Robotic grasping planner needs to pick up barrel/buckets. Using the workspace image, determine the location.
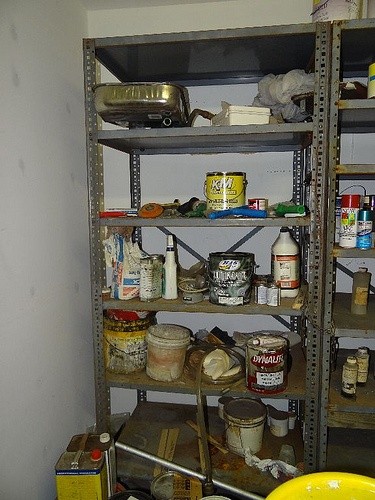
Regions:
[102,317,151,375]
[203,251,256,307]
[145,323,191,382]
[202,170,248,213]
[244,334,289,397]
[55,432,117,500]
[218,396,297,456]
[112,471,232,500]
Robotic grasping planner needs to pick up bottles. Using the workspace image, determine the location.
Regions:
[351,267,371,315]
[341,356,358,398]
[254,281,281,307]
[269,227,300,298]
[355,348,369,388]
[334,194,375,251]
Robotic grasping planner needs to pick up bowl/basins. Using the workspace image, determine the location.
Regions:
[185,345,245,385]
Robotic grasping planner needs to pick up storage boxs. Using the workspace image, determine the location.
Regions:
[212,106,271,125]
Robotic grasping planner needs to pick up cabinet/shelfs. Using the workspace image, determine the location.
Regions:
[82,18,375,500]
